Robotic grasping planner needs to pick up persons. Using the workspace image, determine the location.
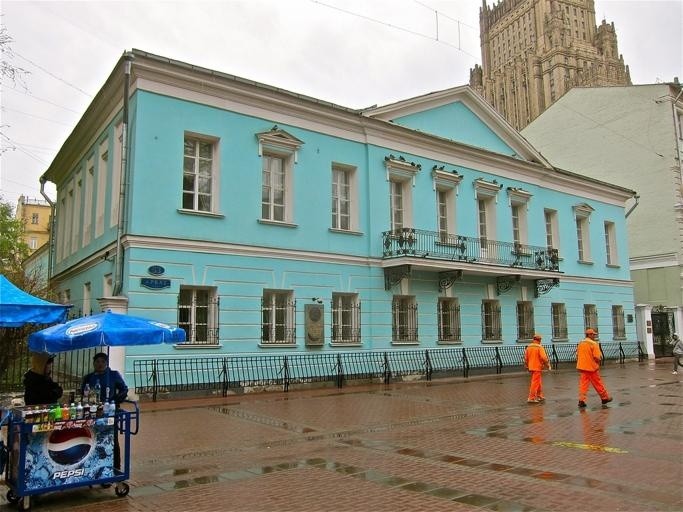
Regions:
[525,335,551,403]
[667,333,683,374]
[24,353,64,405]
[81,352,128,469]
[576,329,614,404]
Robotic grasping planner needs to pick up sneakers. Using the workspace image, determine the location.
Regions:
[537,397,546,401]
[672,371,678,374]
[527,398,540,403]
[602,398,613,404]
[578,401,586,406]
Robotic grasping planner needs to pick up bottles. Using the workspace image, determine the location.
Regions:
[23,398,117,424]
[68,379,102,403]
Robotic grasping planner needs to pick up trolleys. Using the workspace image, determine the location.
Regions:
[0,389,139,512]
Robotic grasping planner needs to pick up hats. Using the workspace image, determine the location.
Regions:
[534,334,543,340]
[93,353,107,361]
[585,329,598,335]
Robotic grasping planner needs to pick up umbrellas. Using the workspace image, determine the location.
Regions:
[28,307,187,354]
